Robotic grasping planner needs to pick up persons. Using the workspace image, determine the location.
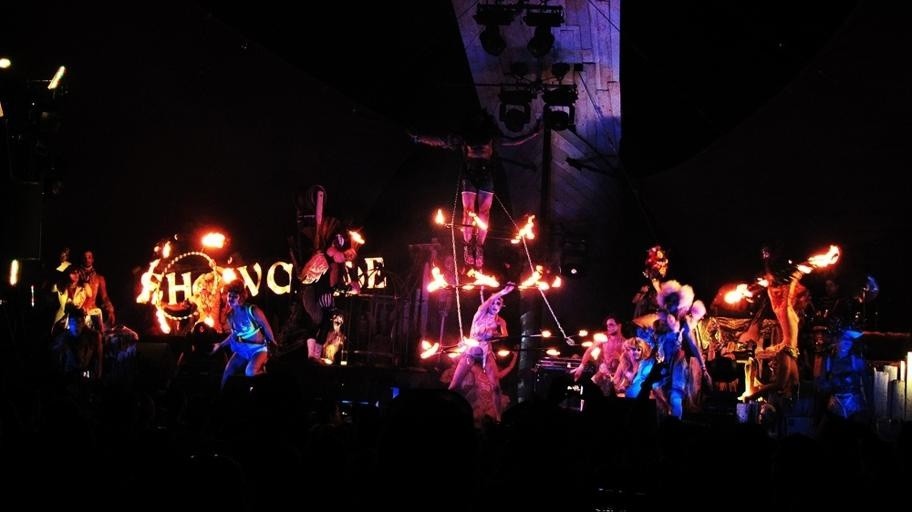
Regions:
[2,225,911,512]
[404,109,542,270]
